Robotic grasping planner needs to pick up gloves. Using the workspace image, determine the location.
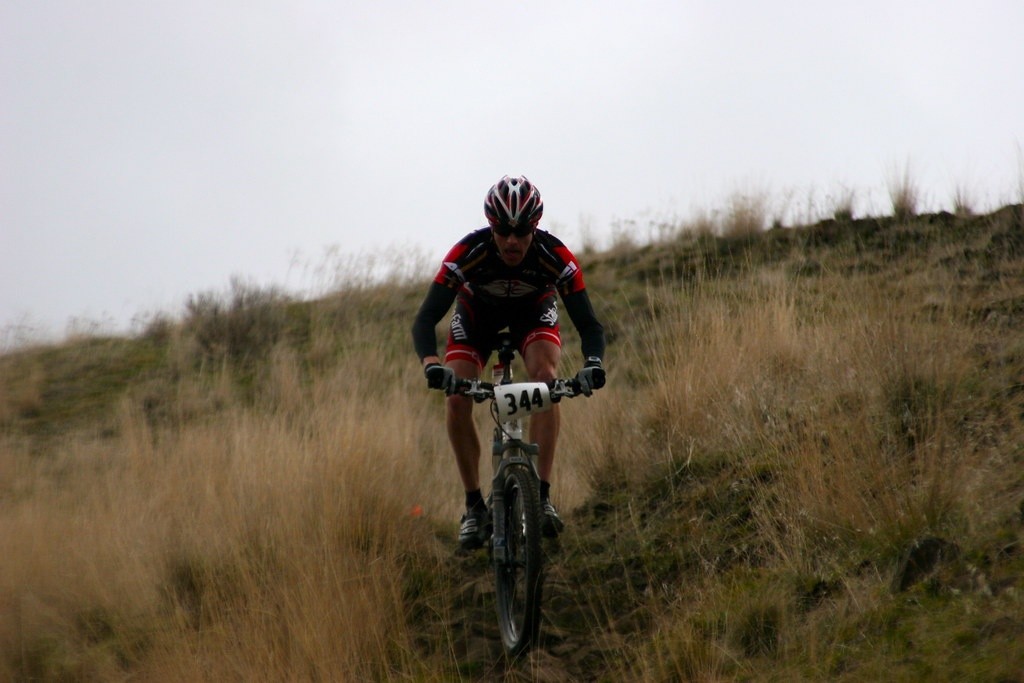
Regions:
[575,360,606,389]
[424,363,450,389]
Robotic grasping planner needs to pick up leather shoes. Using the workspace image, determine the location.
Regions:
[525,498,564,536]
[458,496,491,548]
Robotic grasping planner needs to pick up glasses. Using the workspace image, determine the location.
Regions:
[491,222,535,237]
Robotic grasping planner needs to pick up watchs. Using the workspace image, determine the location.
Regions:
[584,356,602,369]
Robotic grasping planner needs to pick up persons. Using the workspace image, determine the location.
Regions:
[412,175,607,548]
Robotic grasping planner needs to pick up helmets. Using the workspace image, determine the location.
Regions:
[484,175,543,227]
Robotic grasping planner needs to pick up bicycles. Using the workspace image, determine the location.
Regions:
[439,375,584,655]
